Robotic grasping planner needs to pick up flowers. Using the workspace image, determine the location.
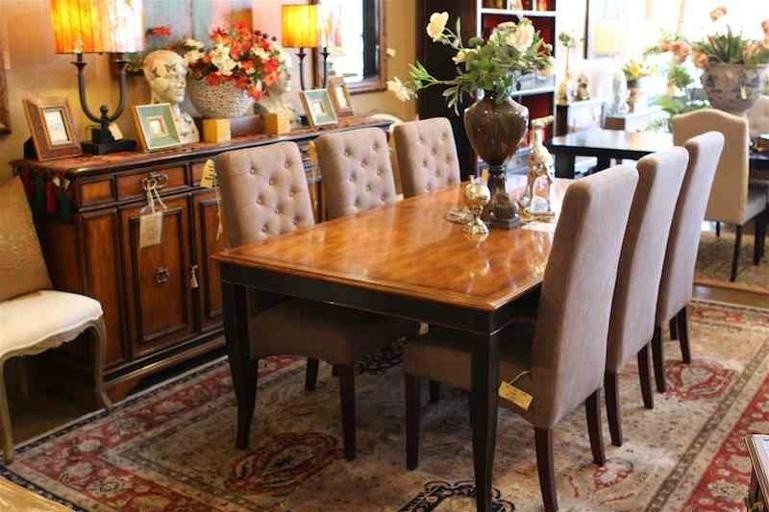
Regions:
[142,22,290,102]
[645,6,769,72]
[386,11,553,116]
[623,61,656,79]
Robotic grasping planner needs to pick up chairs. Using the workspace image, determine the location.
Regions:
[649,130,725,395]
[672,108,767,283]
[600,144,695,447]
[394,115,460,197]
[318,127,397,376]
[1,175,116,468]
[214,140,419,462]
[402,160,644,503]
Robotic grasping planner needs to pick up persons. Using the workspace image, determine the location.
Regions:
[143,49,200,145]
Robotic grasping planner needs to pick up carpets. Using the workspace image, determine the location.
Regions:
[1,276,768,508]
[693,224,769,295]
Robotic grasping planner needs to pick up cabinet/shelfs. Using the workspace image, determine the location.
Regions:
[384,0,555,180]
[9,125,388,390]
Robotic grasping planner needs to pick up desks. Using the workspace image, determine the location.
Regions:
[542,129,769,256]
[556,100,609,136]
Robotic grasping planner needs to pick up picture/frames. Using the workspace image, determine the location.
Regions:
[299,88,338,128]
[22,97,84,162]
[129,102,181,154]
[329,77,353,117]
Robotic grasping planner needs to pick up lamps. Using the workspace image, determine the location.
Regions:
[50,0,145,155]
[281,4,330,89]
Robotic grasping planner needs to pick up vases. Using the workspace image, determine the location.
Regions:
[699,61,762,116]
[463,86,529,232]
